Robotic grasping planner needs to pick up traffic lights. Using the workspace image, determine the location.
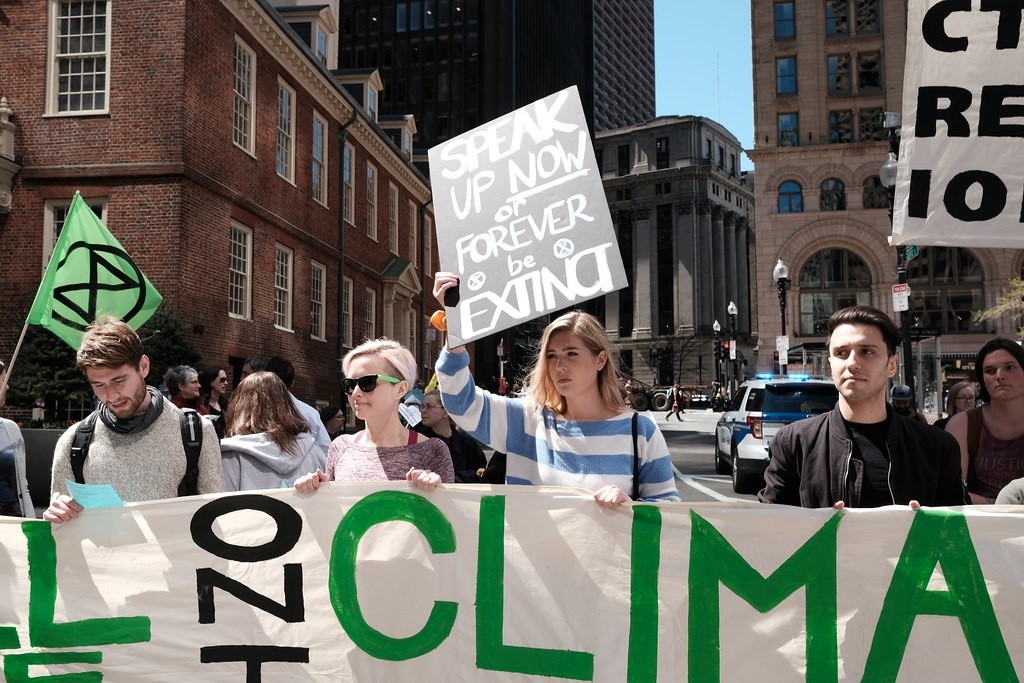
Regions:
[723,342,729,359]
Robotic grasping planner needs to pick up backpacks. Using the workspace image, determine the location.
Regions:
[667,387,673,401]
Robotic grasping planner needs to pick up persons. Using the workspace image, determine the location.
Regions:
[159,352,506,484]
[294,335,455,492]
[0,359,38,518]
[618,377,636,410]
[890,378,978,430]
[432,270,681,509]
[43,312,226,523]
[945,337,1024,505]
[220,371,327,492]
[711,377,725,404]
[665,381,686,422]
[758,305,965,508]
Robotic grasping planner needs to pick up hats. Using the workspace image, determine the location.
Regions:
[320,405,340,424]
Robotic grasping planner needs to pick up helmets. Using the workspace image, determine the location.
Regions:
[892,385,912,400]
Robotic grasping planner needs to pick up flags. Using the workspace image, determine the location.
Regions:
[26,190,164,352]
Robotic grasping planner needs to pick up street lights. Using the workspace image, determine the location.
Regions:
[880,128,918,413]
[728,301,738,394]
[713,320,722,389]
[773,258,791,375]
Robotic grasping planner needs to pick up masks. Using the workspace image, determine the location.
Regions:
[893,399,911,417]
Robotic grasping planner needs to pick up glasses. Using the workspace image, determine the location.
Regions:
[954,397,975,403]
[216,377,228,383]
[419,403,442,412]
[341,374,401,396]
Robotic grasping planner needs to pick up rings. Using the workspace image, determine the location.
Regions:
[427,475,431,480]
[420,469,423,474]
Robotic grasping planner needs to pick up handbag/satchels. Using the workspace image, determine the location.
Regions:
[672,402,678,412]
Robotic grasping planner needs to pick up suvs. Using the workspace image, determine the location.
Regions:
[715,379,841,493]
